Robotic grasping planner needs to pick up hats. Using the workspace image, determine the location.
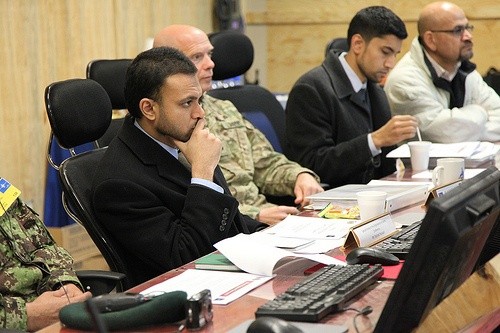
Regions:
[58,291,190,333]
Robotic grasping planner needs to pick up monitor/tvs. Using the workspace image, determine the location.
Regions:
[371,165,500,333]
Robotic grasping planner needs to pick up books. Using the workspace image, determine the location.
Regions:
[195,253,242,271]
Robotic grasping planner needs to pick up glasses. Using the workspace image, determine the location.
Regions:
[431,25,474,38]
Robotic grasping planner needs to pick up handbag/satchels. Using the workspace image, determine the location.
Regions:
[483,67,500,95]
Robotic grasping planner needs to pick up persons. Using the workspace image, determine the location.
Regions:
[92,46,270,285]
[0,177,92,333]
[383,2,500,143]
[285,6,418,191]
[153,24,324,226]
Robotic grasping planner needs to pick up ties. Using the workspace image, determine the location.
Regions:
[358,88,368,106]
[177,150,192,172]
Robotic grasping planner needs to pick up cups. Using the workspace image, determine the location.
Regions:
[432,158,464,187]
[356,191,387,222]
[407,141,432,170]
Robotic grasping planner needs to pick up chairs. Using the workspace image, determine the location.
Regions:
[43,30,348,298]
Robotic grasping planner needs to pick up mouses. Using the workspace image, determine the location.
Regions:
[346,247,399,265]
[246,316,302,333]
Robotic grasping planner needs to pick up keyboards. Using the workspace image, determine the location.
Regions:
[255,264,384,321]
[372,219,423,259]
